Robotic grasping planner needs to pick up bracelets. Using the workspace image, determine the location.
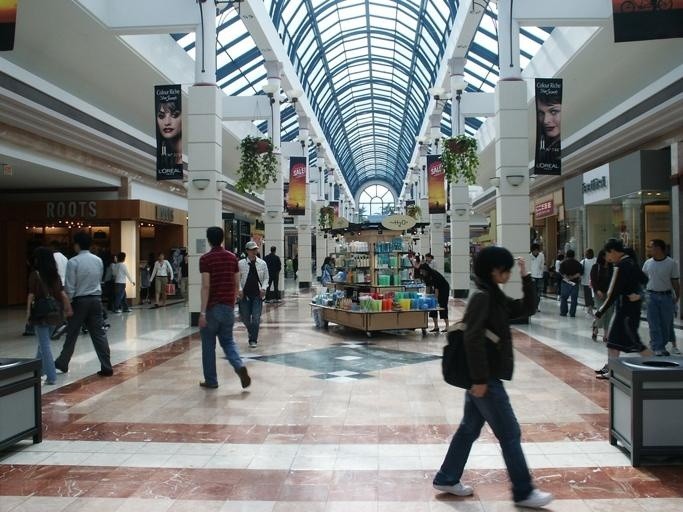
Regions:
[201,312,206,316]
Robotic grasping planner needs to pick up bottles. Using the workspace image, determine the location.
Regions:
[349,254,369,283]
[340,297,352,310]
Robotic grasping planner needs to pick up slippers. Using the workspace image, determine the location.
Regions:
[595,373,608,379]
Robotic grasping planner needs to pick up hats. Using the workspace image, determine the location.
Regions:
[245,241,258,250]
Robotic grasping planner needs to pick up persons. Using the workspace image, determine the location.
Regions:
[237,241,269,347]
[580,249,597,306]
[641,239,677,354]
[403,250,450,332]
[537,97,560,151]
[149,252,173,306]
[156,102,182,164]
[26,248,72,384]
[286,255,298,280]
[556,249,583,317]
[198,227,251,389]
[264,246,282,303]
[530,244,545,312]
[432,246,553,509]
[321,257,346,292]
[590,238,652,378]
[51,230,135,377]
[178,253,188,306]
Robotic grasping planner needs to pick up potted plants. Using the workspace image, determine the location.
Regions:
[231,132,278,195]
[404,203,420,222]
[436,132,481,187]
[316,204,336,232]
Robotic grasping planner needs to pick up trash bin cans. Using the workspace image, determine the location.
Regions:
[0,358,42,449]
[607,358,683,467]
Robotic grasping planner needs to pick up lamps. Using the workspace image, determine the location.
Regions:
[506,174,524,186]
[260,81,279,142]
[297,133,343,189]
[453,81,468,130]
[489,176,500,187]
[279,89,302,114]
[403,132,441,189]
[216,180,227,192]
[191,178,208,189]
[428,87,452,111]
[529,177,536,186]
[267,210,278,220]
[182,180,188,190]
[455,209,465,215]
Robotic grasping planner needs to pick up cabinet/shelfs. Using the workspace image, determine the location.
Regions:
[312,242,445,340]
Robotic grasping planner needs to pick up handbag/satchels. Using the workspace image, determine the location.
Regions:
[29,270,62,321]
[588,263,600,288]
[164,284,175,295]
[441,290,503,391]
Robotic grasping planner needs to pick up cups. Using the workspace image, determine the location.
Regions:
[327,299,333,306]
[372,300,382,312]
[399,299,410,310]
[385,242,389,252]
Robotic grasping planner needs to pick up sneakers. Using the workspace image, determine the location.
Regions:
[249,341,257,347]
[199,380,217,388]
[239,366,250,388]
[670,346,679,354]
[433,479,474,496]
[513,487,554,508]
